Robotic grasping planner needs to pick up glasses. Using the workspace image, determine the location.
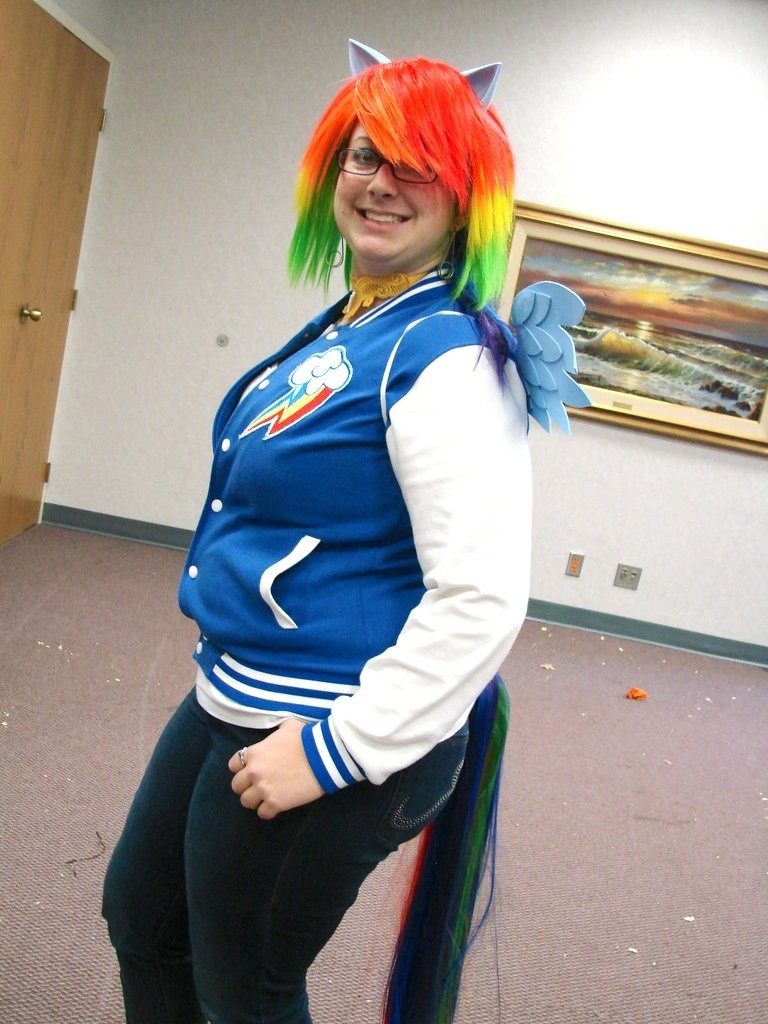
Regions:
[338,148,437,184]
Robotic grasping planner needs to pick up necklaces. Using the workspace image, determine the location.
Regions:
[341,270,429,321]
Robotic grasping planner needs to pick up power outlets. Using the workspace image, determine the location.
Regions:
[613,563,643,591]
[565,551,585,577]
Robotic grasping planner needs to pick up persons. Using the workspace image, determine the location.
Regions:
[94,55,537,1023]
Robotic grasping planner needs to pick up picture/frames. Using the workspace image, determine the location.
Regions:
[488,198,768,459]
